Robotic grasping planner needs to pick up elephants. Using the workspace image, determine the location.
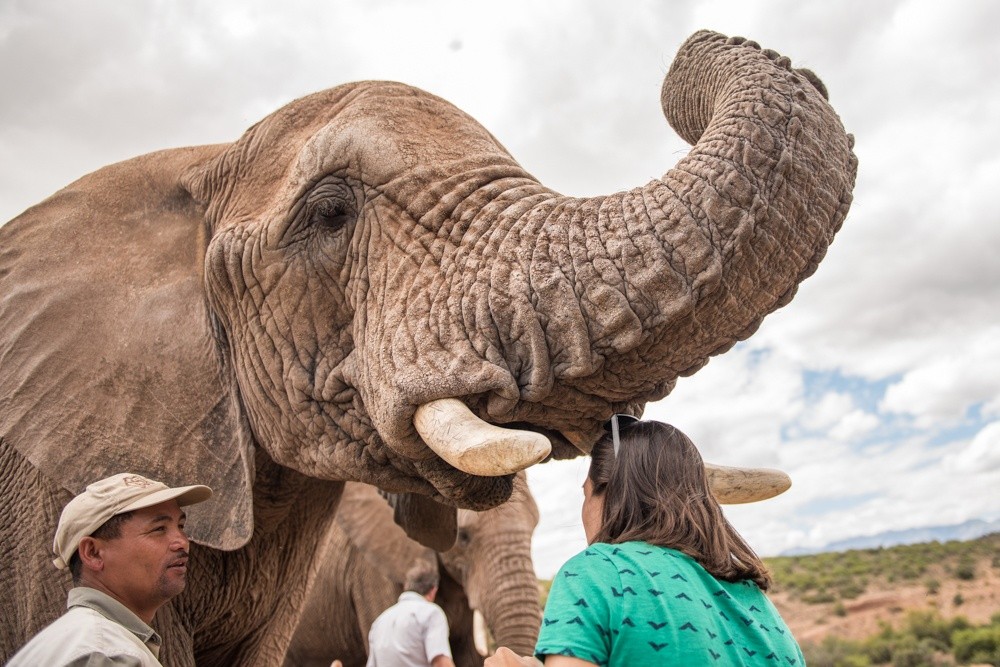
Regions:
[0,29,859,667]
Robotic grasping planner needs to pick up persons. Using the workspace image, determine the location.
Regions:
[366,564,455,667]
[0,473,215,667]
[485,414,804,667]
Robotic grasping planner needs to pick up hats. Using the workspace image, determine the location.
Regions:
[53,470,214,570]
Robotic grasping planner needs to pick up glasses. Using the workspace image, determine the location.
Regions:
[602,413,640,460]
